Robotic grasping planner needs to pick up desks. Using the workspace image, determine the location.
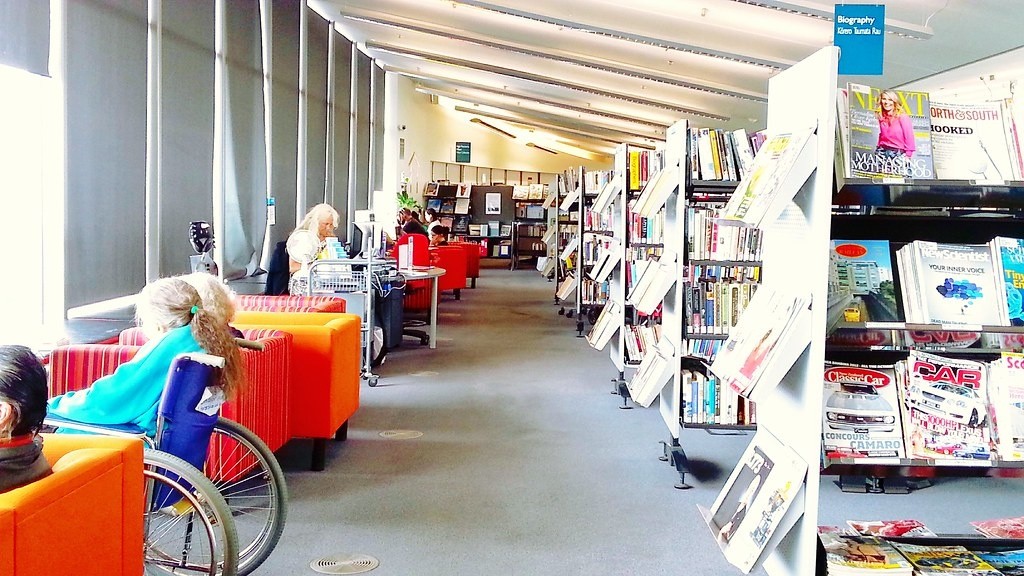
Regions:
[396,266,447,350]
[306,239,447,386]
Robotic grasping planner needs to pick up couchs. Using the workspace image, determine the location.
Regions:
[453,243,481,290]
[391,235,440,311]
[428,245,466,299]
[236,294,346,313]
[228,311,362,472]
[0,433,143,576]
[48,326,293,514]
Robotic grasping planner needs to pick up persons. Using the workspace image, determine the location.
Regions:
[0,345,51,491]
[285,203,340,295]
[871,89,916,176]
[397,201,450,247]
[36,272,245,446]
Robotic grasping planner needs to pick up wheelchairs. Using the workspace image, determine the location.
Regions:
[38,337,289,576]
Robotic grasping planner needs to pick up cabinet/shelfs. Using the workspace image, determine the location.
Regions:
[415,48,1024,576]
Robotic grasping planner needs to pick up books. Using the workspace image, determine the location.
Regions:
[424,173,550,259]
[630,118,772,426]
[553,164,614,308]
[817,83,1024,576]
